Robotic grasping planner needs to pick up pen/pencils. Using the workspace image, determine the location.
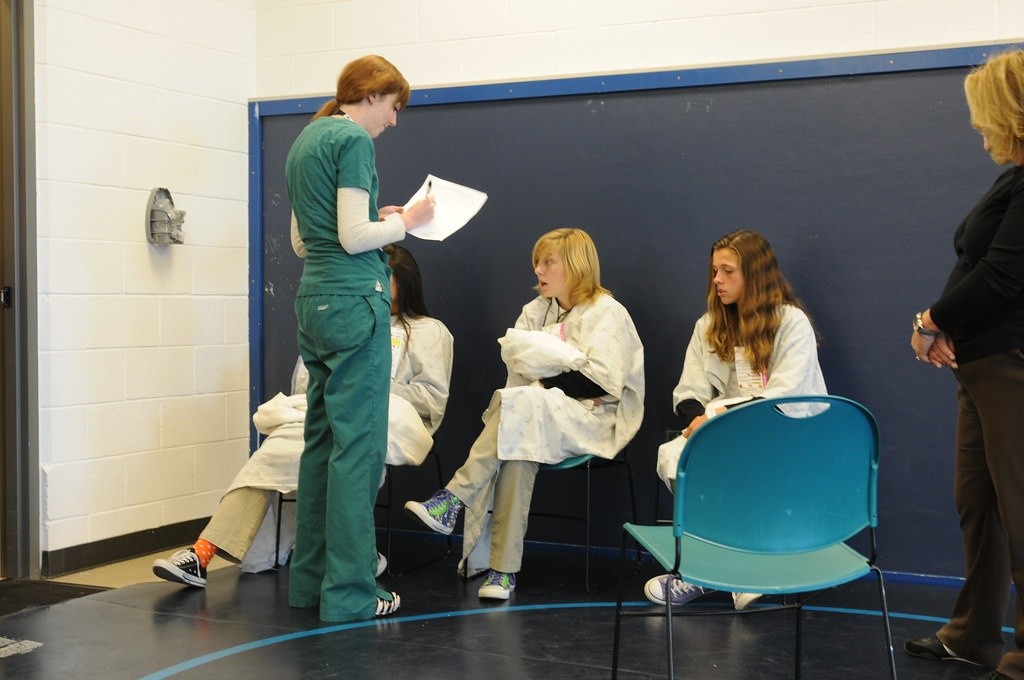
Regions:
[425,180,433,199]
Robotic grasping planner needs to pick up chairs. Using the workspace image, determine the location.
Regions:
[612,396,901,680]
[464,446,643,594]
[274,451,452,578]
[652,429,831,606]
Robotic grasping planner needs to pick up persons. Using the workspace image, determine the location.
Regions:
[644,228,830,609]
[901,50,1024,680]
[404,228,645,599]
[284,54,437,622]
[154,244,454,588]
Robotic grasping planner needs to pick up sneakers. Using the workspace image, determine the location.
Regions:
[477,570,516,600]
[374,551,387,578]
[644,574,716,606]
[978,671,1012,680]
[374,591,400,616]
[152,548,207,589]
[732,591,763,611]
[904,636,987,667]
[404,489,465,536]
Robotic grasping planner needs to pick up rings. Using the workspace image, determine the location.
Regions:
[916,356,920,360]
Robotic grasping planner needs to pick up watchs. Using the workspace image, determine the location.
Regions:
[913,312,936,335]
